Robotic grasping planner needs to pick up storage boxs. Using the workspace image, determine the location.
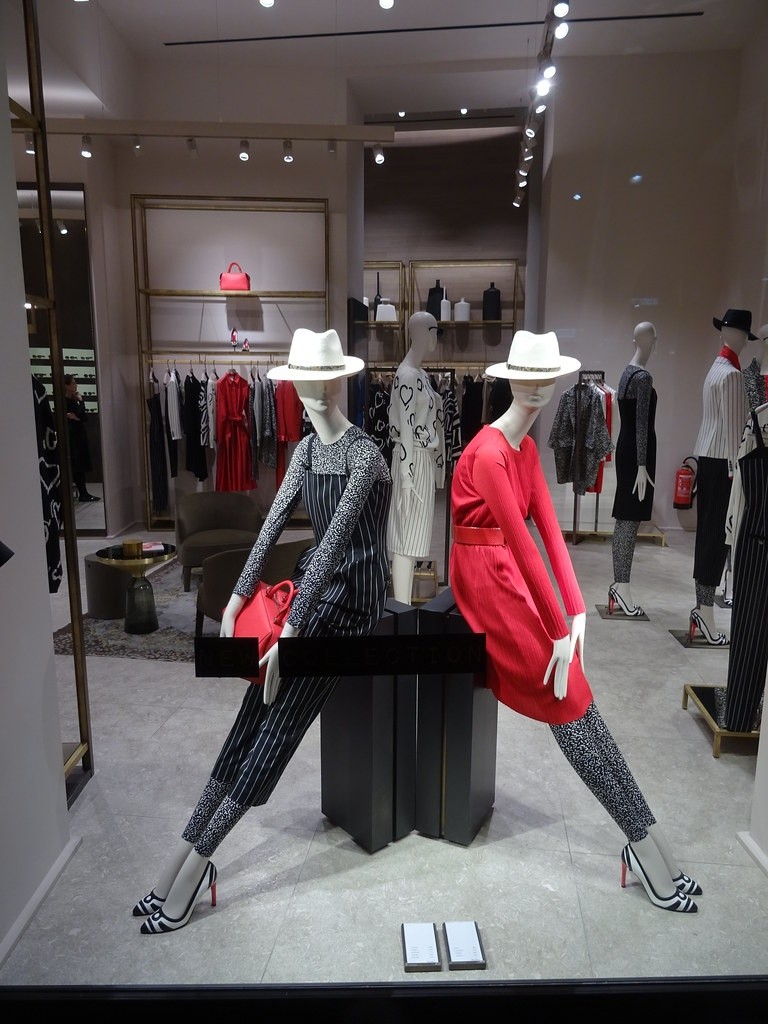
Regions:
[417,582,500,845]
[314,594,413,853]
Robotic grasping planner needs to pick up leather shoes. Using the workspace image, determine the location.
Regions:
[78,494,101,502]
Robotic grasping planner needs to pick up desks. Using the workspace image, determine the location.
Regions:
[98,541,179,636]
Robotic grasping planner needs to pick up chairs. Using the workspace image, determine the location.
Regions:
[191,534,321,638]
[172,489,262,589]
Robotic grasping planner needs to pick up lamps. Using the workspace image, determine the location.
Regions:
[80,135,94,160]
[282,140,294,164]
[550,0,571,19]
[35,220,41,234]
[518,158,534,176]
[522,116,544,138]
[536,51,557,79]
[544,14,570,40]
[513,190,526,208]
[328,140,338,154]
[238,139,250,163]
[23,133,34,156]
[514,169,528,188]
[521,130,539,149]
[520,141,534,161]
[530,79,555,98]
[57,220,68,236]
[186,138,197,155]
[528,88,548,115]
[130,135,147,158]
[373,143,385,167]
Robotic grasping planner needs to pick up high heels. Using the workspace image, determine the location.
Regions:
[609,588,645,616]
[723,569,734,606]
[688,606,730,645]
[230,328,250,352]
[619,844,703,913]
[133,861,217,934]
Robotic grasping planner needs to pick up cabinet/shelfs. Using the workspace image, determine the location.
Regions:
[28,339,99,417]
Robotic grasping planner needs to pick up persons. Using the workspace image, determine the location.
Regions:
[65,374,101,502]
[743,324,768,411]
[450,329,703,913]
[689,308,760,645]
[132,328,394,935]
[608,322,658,616]
[387,312,445,605]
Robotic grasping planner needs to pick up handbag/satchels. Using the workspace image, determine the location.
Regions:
[219,262,251,291]
[221,579,301,685]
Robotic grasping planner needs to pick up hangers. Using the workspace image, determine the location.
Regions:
[371,363,503,383]
[572,370,607,393]
[147,358,286,381]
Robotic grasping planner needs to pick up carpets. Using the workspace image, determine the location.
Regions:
[52,557,222,664]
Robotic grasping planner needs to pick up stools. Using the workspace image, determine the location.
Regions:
[86,552,132,619]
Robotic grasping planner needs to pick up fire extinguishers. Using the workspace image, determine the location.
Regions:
[672,457,700,509]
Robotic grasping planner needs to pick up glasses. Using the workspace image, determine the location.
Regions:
[33,355,44,358]
[428,326,444,337]
[66,374,78,376]
[65,356,77,359]
[83,391,95,395]
[34,374,46,377]
[81,357,92,359]
[84,374,95,377]
[85,409,96,412]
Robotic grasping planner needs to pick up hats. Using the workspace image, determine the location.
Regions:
[484,331,581,381]
[713,309,760,341]
[266,328,365,381]
